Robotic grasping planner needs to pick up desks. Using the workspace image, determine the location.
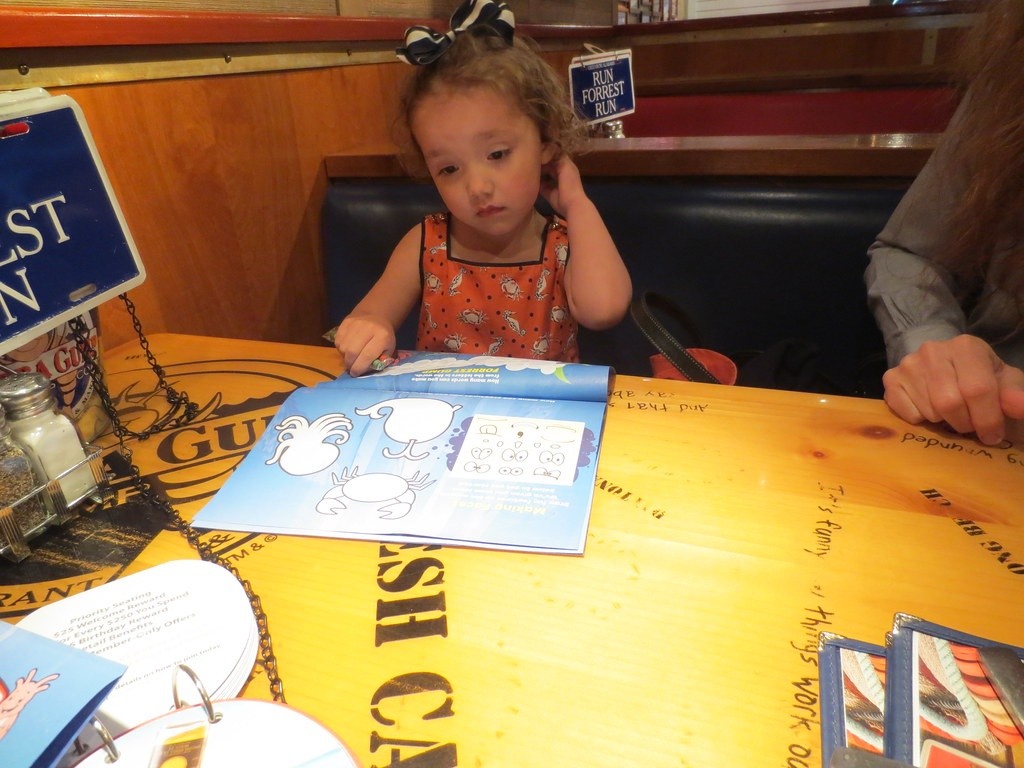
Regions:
[0,334,1024,768]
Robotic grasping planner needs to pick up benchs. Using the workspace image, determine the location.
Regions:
[317,129,992,400]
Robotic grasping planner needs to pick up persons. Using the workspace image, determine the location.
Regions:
[335,0,633,364]
[861,0,1024,446]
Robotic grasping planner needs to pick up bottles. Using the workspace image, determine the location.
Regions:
[605,119,625,139]
[0,373,96,542]
